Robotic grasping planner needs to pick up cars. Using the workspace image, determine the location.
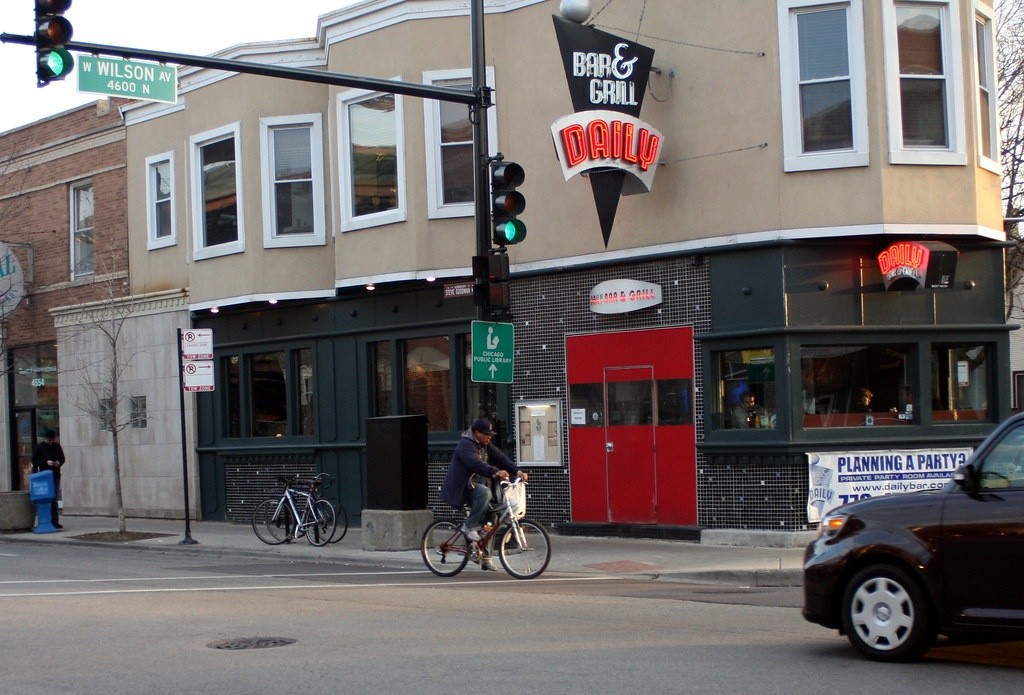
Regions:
[802,412,1024,664]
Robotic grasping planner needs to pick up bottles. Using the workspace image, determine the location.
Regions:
[480,522,492,538]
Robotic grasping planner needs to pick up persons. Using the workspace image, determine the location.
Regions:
[847,390,873,414]
[438,420,530,571]
[31,430,66,529]
[900,387,913,414]
[732,393,757,429]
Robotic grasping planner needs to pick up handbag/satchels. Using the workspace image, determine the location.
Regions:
[502,481,527,524]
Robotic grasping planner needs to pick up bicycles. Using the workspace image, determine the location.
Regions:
[421,475,552,580]
[251,472,349,547]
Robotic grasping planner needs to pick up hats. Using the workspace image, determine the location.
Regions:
[474,418,497,436]
[44,430,57,439]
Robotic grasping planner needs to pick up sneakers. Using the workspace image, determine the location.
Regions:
[461,523,481,542]
[481,560,498,571]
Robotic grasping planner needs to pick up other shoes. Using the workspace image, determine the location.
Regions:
[54,523,63,529]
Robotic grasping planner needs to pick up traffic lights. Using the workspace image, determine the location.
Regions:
[472,251,511,315]
[35,0,75,81]
[491,162,527,246]
